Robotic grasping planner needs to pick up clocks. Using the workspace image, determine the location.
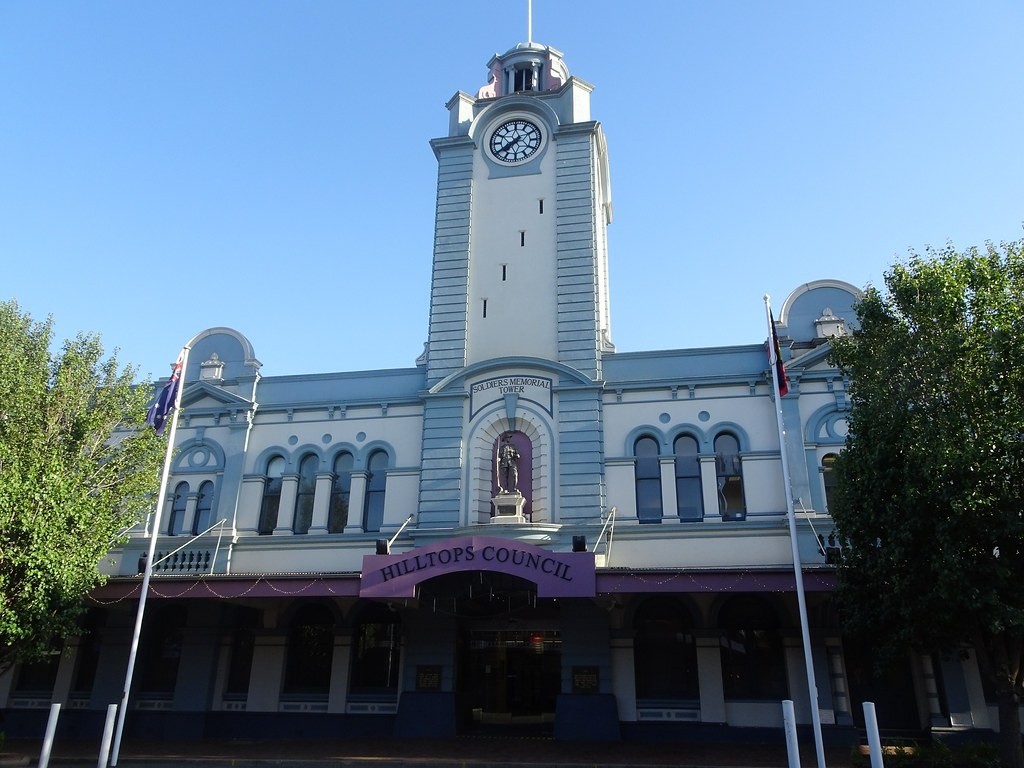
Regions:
[482,111,548,167]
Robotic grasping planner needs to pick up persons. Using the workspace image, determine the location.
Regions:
[496,433,520,493]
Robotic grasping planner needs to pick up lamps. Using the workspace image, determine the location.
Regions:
[376,516,412,556]
[572,536,587,552]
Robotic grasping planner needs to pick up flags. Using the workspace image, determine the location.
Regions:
[147,362,183,437]
[768,309,788,397]
